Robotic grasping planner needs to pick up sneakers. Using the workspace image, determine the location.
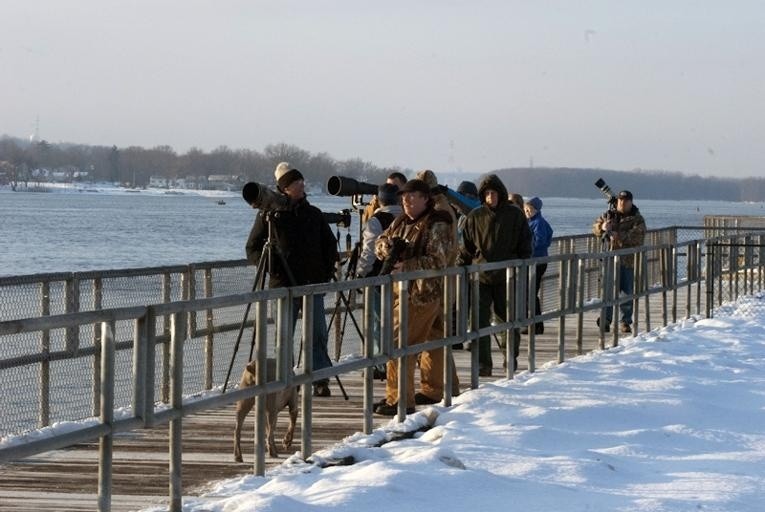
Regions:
[416,393,438,405]
[522,327,544,335]
[375,405,415,415]
[479,369,492,376]
[596,318,610,332]
[312,385,332,398]
[621,323,632,333]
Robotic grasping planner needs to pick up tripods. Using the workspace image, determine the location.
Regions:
[218,240,380,400]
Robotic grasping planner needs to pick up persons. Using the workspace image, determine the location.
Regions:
[243,160,338,398]
[349,168,554,378]
[370,179,455,417]
[591,188,647,336]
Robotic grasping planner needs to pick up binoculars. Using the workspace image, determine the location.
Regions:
[220,182,353,399]
[594,178,618,241]
[327,176,385,397]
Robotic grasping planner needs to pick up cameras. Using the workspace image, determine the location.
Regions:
[594,176,616,202]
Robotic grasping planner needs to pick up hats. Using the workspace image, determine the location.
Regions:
[414,170,438,188]
[526,197,543,211]
[274,161,305,190]
[456,181,478,195]
[396,179,429,195]
[615,190,634,201]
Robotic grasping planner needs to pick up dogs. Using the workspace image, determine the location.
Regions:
[233,358,298,463]
